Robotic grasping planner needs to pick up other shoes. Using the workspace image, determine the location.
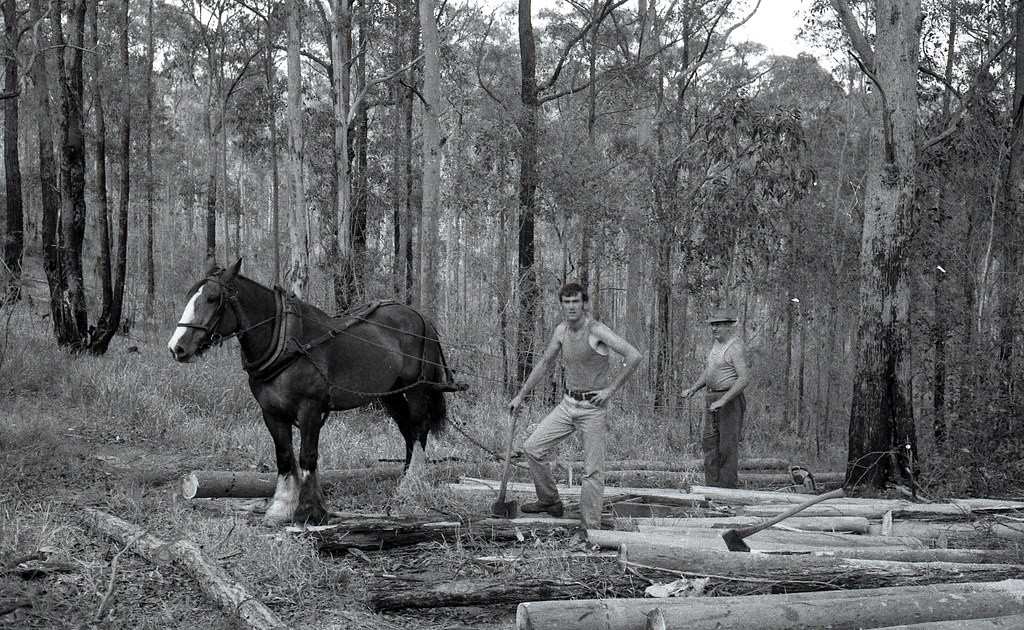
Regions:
[521,500,564,518]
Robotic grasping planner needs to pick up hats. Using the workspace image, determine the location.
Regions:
[705,309,737,326]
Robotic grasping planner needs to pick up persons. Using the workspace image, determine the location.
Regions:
[509,282,643,540]
[680,317,750,489]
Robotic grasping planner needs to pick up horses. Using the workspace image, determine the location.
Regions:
[166,256,447,527]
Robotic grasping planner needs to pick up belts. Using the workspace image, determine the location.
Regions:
[565,388,598,401]
[707,388,730,394]
